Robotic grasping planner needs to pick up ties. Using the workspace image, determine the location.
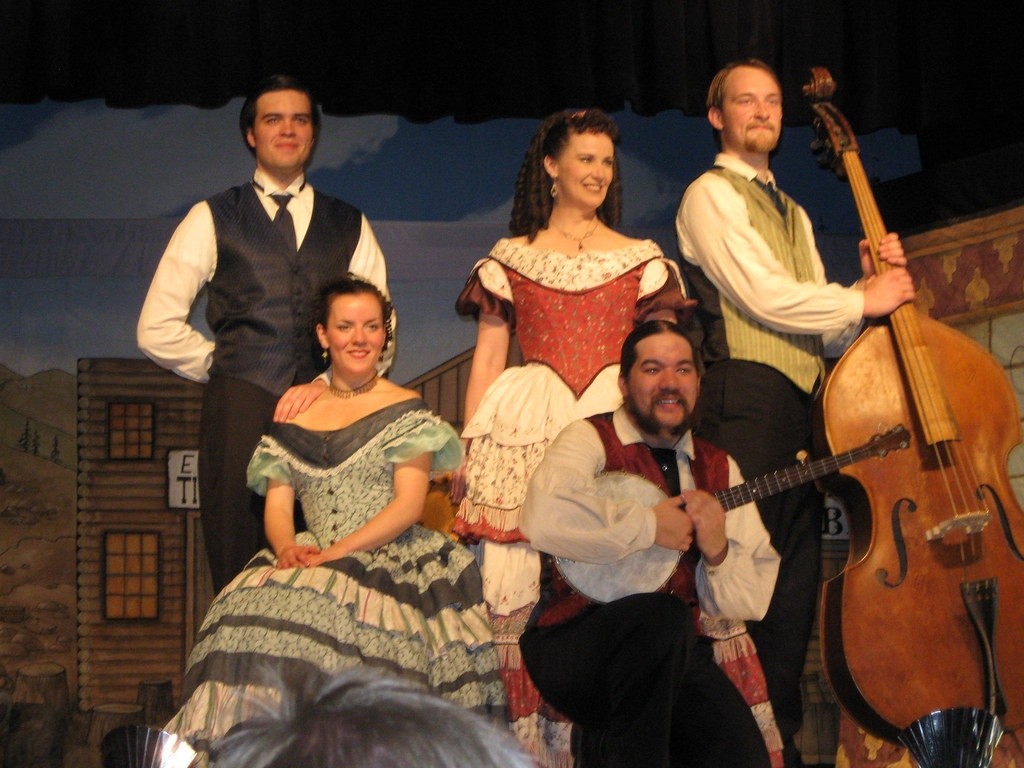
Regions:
[708,161,788,218]
[251,171,307,255]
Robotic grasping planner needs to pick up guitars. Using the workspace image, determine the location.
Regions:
[547,421,916,608]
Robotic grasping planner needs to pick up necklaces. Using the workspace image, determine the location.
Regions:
[549,215,600,250]
[330,370,379,399]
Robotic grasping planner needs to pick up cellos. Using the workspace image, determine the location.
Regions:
[799,58,1024,746]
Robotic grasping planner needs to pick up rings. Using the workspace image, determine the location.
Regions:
[680,493,688,503]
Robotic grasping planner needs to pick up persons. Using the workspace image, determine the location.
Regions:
[162,273,507,768]
[208,661,534,768]
[675,59,916,768]
[519,321,782,768]
[137,73,395,595]
[450,107,783,768]
